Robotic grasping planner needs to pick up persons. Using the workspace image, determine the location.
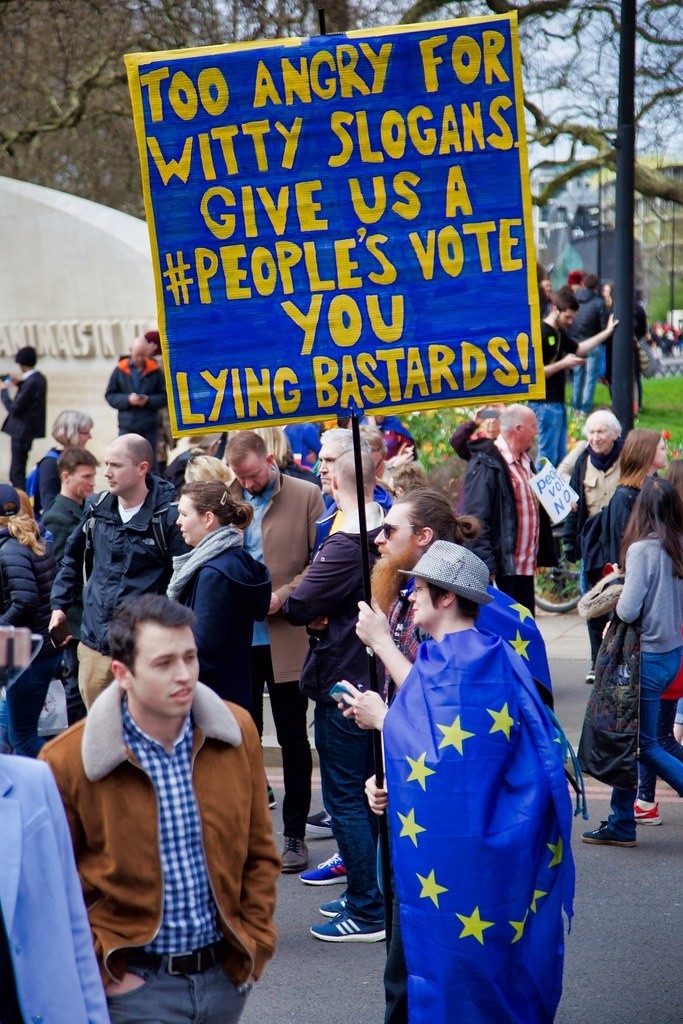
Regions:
[0,407,550,942]
[647,322,683,357]
[0,346,47,489]
[0,752,110,1024]
[338,540,590,1023]
[38,594,282,1024]
[536,262,648,468]
[106,331,176,476]
[557,409,683,847]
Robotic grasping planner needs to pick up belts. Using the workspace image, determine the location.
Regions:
[124,941,229,976]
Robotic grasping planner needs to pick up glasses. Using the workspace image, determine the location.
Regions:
[380,524,416,540]
[412,584,430,592]
[657,445,670,453]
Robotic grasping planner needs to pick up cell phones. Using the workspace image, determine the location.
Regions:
[328,682,355,707]
[480,410,499,419]
[0,628,33,669]
[49,620,71,649]
[138,394,144,399]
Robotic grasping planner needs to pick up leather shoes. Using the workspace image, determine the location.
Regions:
[280,837,309,874]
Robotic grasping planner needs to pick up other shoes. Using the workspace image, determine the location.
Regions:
[266,786,277,809]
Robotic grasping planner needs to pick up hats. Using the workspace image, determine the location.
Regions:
[144,330,163,357]
[0,483,21,516]
[15,346,37,368]
[396,540,496,606]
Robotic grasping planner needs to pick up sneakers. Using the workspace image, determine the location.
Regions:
[310,910,387,943]
[305,809,333,836]
[298,851,348,885]
[319,896,348,918]
[633,798,662,826]
[580,820,638,847]
[585,663,596,684]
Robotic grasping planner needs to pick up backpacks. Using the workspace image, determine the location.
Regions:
[25,451,60,520]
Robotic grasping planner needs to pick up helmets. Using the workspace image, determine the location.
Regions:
[567,269,588,286]
